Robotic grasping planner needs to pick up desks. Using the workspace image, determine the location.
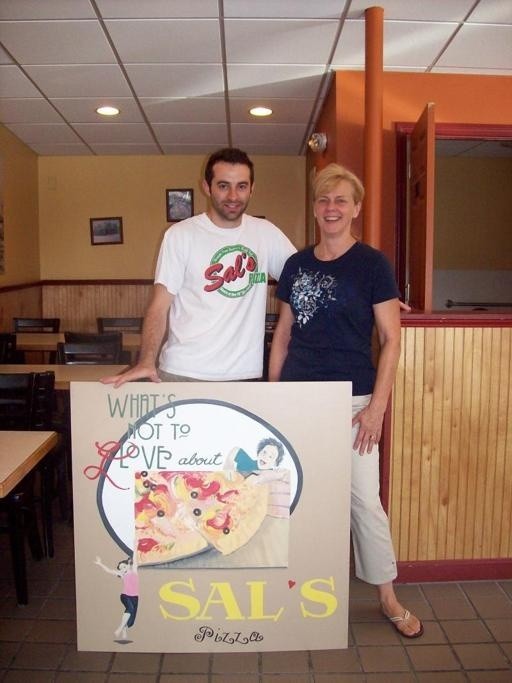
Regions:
[0,428,60,609]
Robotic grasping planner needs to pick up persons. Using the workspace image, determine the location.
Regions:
[268,161,424,642]
[93,535,140,640]
[221,437,284,482]
[99,145,415,390]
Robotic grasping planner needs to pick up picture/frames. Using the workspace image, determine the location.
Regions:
[89,216,123,245]
[165,188,195,222]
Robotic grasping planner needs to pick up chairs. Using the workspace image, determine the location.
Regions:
[1,316,145,560]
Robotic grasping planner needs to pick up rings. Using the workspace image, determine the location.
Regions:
[369,434,377,439]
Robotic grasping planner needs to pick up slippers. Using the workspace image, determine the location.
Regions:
[385,609,424,639]
